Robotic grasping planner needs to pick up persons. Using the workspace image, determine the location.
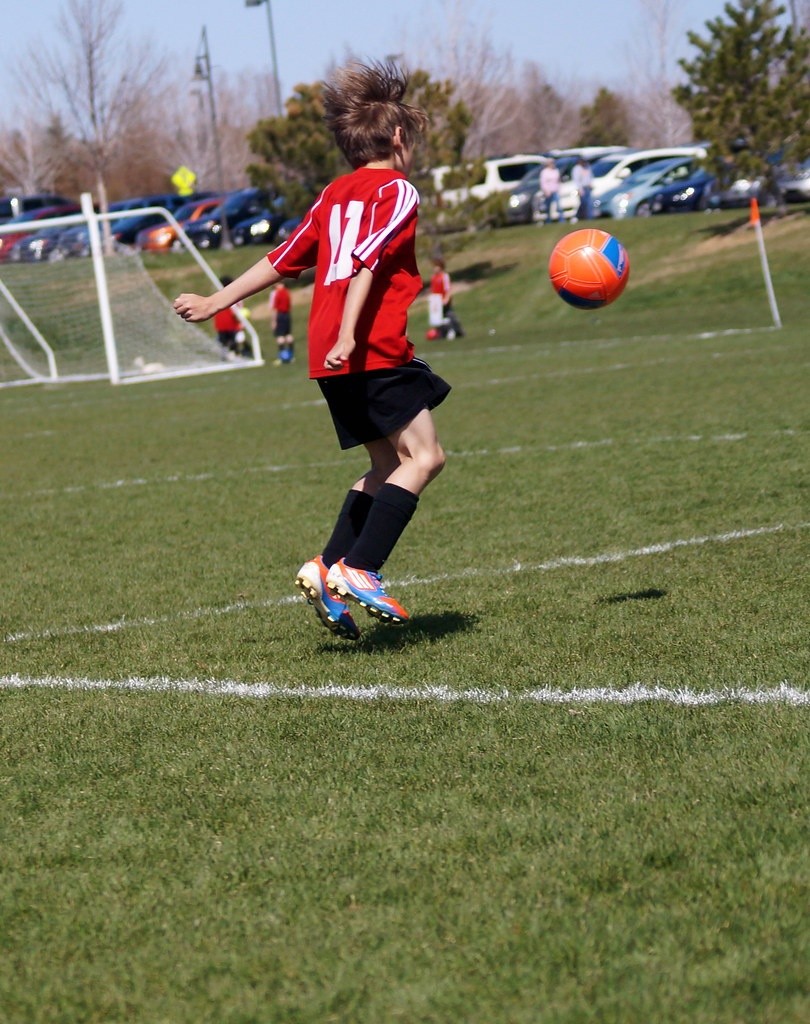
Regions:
[171,57,452,638]
[269,282,299,368]
[425,258,457,341]
[571,157,594,220]
[213,277,247,360]
[539,158,566,223]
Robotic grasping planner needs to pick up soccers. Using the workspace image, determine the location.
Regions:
[549,228,630,311]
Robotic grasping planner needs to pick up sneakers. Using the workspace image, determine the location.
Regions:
[295,554,359,641]
[325,557,409,625]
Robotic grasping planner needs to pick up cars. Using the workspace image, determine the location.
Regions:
[0,186,302,262]
[410,141,810,219]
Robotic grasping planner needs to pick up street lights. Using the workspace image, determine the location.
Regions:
[192,25,234,251]
[246,0,282,117]
